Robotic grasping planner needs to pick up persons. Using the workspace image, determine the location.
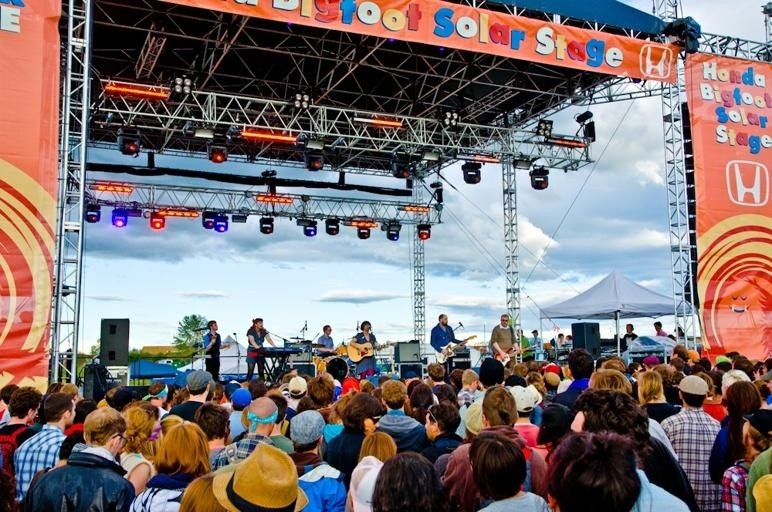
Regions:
[128,421,212,512]
[59,383,81,401]
[668,357,684,372]
[287,375,307,409]
[490,313,523,379]
[636,371,682,425]
[653,320,668,336]
[660,375,722,512]
[224,381,242,413]
[349,320,384,381]
[305,374,335,411]
[628,361,645,377]
[720,411,772,512]
[366,452,449,512]
[697,355,724,395]
[517,329,533,363]
[97,384,133,411]
[746,445,772,511]
[245,317,277,386]
[287,409,347,512]
[194,402,233,460]
[179,473,227,512]
[323,391,388,492]
[171,367,213,422]
[504,361,529,389]
[570,388,654,470]
[697,371,728,422]
[25,429,86,512]
[444,385,550,512]
[425,403,465,463]
[201,319,231,383]
[45,383,63,393]
[148,381,169,419]
[543,370,561,405]
[565,335,573,345]
[374,379,428,450]
[467,431,551,511]
[326,357,350,402]
[316,324,338,358]
[708,381,771,486]
[429,313,467,378]
[622,323,638,350]
[122,400,156,419]
[0,385,43,512]
[509,384,544,447]
[210,397,277,474]
[671,344,689,365]
[0,383,19,424]
[555,332,571,351]
[588,368,679,462]
[349,430,398,512]
[528,329,542,360]
[211,383,227,402]
[673,326,685,339]
[64,398,98,436]
[120,406,155,512]
[731,357,754,381]
[13,392,77,512]
[536,402,575,446]
[227,387,253,440]
[544,429,691,512]
[641,355,661,369]
[28,407,136,512]
[549,348,596,402]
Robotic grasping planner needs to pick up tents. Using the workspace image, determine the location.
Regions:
[538,267,698,362]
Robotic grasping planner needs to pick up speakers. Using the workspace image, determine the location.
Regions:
[571,321,602,362]
[99,318,130,367]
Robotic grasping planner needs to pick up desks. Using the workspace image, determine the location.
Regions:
[256,347,301,383]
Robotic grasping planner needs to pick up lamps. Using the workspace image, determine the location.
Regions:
[308,155,325,170]
[119,134,141,155]
[512,158,532,170]
[172,77,193,97]
[303,222,317,236]
[392,158,410,177]
[84,204,101,223]
[417,224,432,240]
[358,227,371,240]
[462,162,481,183]
[260,218,274,233]
[536,120,554,136]
[384,221,401,240]
[150,212,166,229]
[325,219,340,235]
[442,109,457,128]
[208,144,228,163]
[112,209,127,225]
[530,167,550,191]
[292,92,311,109]
[201,212,217,230]
[214,216,228,231]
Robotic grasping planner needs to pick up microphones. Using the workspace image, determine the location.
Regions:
[232,332,242,344]
[457,321,464,327]
[259,328,269,334]
[368,325,373,334]
[304,318,308,331]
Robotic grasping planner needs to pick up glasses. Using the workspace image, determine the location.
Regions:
[502,319,508,322]
[370,417,380,425]
[113,433,128,444]
[425,404,435,420]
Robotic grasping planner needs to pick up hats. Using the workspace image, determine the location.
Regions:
[511,384,543,413]
[742,409,772,440]
[544,362,561,377]
[643,357,661,365]
[229,389,252,407]
[672,375,709,395]
[288,375,308,396]
[688,350,701,364]
[349,455,384,512]
[289,410,326,443]
[716,354,733,365]
[761,369,772,380]
[210,444,310,512]
[465,400,483,435]
[545,372,560,386]
[185,368,212,391]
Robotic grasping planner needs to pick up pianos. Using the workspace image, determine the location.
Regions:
[254,346,303,358]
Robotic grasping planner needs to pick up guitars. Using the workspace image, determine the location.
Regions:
[347,340,391,363]
[435,335,477,364]
[494,344,537,368]
